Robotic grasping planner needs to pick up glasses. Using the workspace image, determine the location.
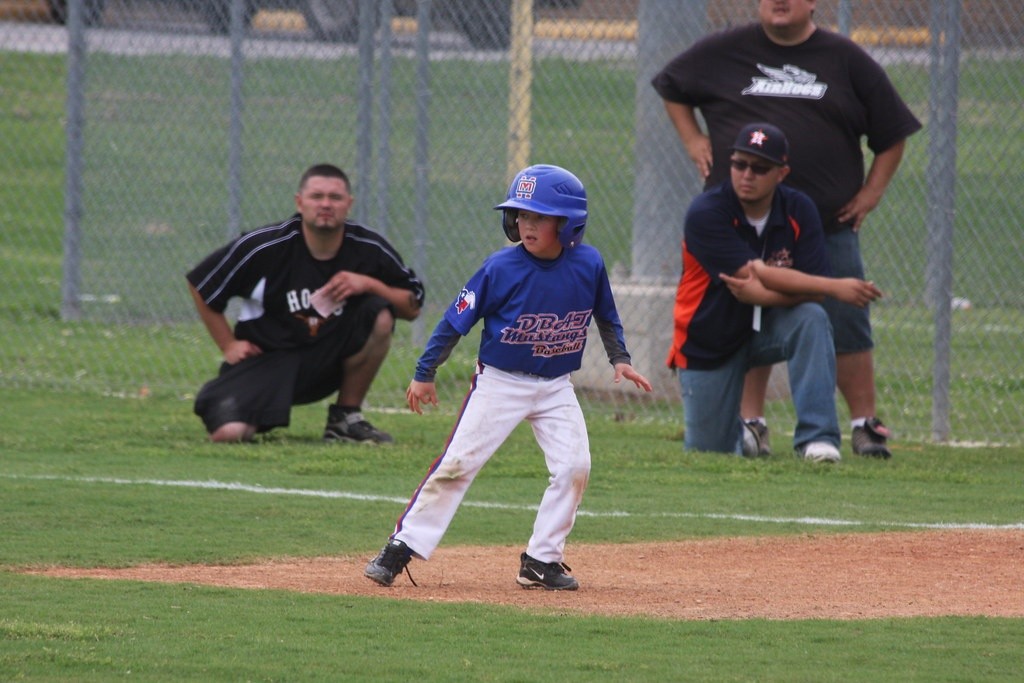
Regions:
[730,155,776,174]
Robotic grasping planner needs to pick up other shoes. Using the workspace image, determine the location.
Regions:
[851,417,892,458]
[747,419,771,456]
[800,441,841,465]
[739,415,761,458]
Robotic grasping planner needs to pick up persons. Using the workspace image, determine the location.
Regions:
[187,165,423,444]
[364,163,649,589]
[651,0,923,460]
[665,125,883,463]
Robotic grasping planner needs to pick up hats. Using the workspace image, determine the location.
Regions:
[732,124,790,166]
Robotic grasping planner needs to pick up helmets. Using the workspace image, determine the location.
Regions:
[493,164,588,249]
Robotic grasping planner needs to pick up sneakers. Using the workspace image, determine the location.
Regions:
[364,537,418,587]
[516,552,581,591]
[323,403,394,449]
